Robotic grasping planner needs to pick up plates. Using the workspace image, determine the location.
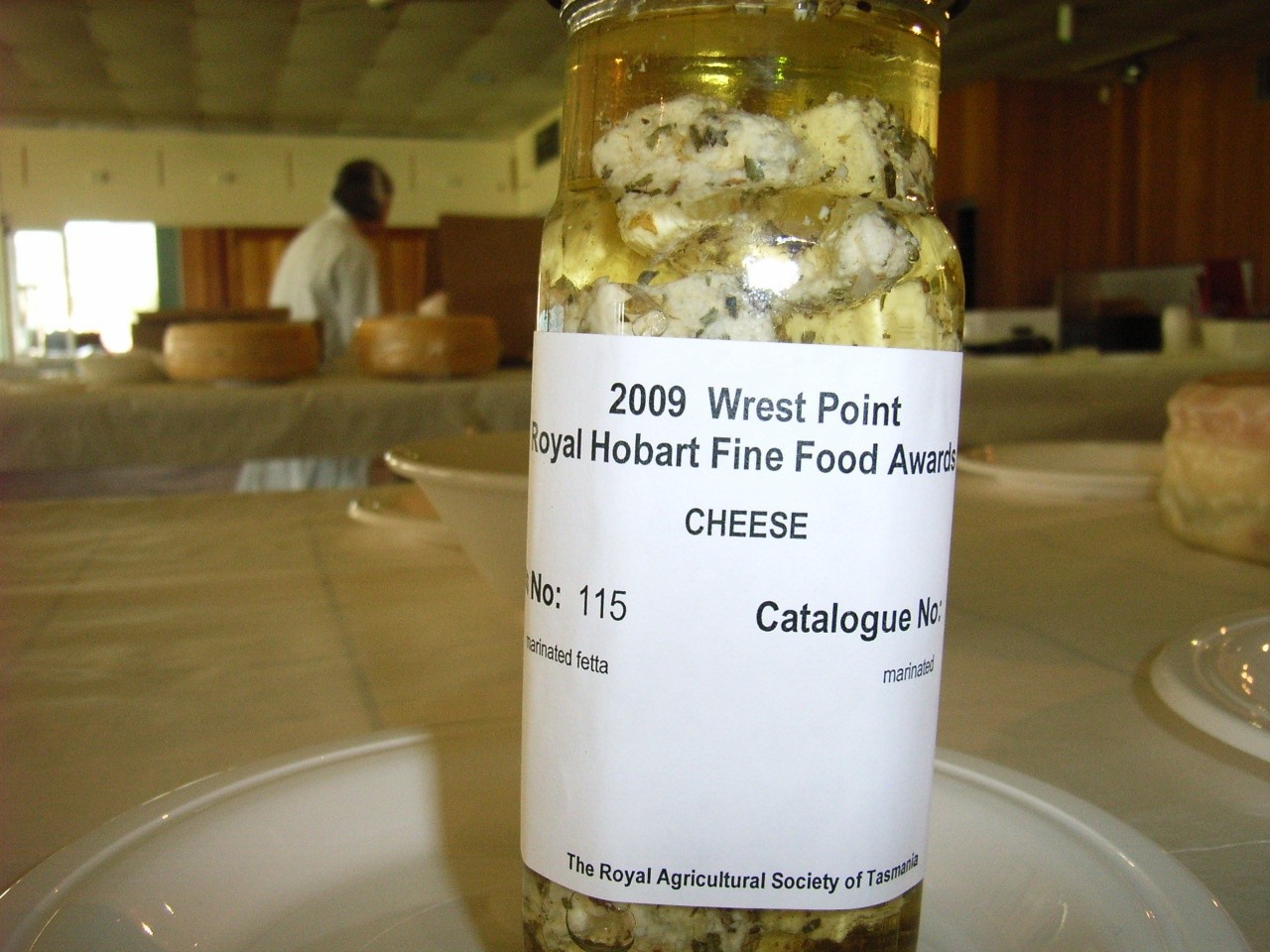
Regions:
[0,713,1250,952]
[955,440,1161,500]
[347,483,459,545]
[1149,606,1270,763]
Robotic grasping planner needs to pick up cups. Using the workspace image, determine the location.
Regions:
[1163,307,1192,352]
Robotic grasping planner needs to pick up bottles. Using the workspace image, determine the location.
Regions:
[517,1,967,952]
[21,330,47,380]
[76,334,112,383]
[42,332,78,380]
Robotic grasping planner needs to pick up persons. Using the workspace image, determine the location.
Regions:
[235,159,393,491]
[416,283,471,319]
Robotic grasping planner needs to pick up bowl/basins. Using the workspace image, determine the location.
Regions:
[163,322,325,383]
[360,317,501,381]
[386,433,529,597]
[1202,320,1270,368]
